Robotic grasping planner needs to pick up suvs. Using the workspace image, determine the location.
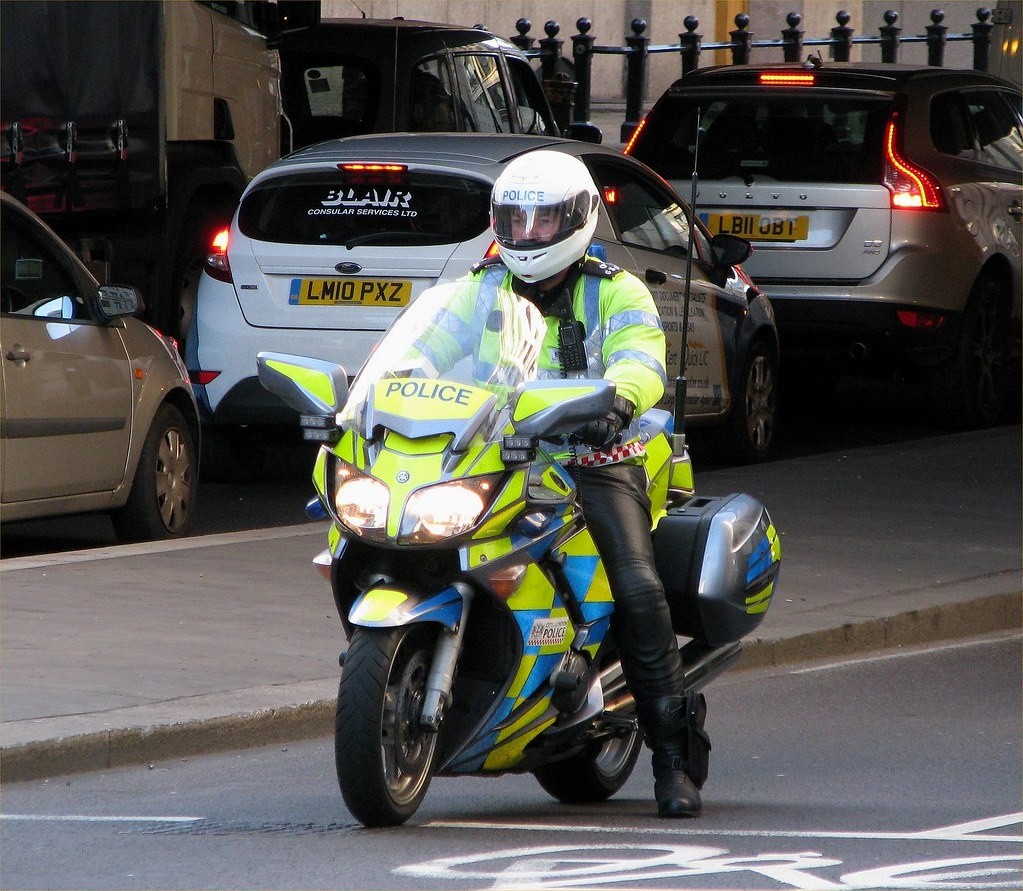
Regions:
[611,57,1023,431]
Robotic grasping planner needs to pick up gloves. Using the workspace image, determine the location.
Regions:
[568,394,636,452]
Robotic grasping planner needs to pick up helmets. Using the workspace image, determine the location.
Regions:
[489,149,600,284]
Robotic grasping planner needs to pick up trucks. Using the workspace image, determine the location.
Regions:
[1,1,294,322]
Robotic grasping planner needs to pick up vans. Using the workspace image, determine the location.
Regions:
[274,16,607,160]
[1,187,202,547]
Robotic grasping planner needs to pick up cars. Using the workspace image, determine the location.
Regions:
[186,130,783,491]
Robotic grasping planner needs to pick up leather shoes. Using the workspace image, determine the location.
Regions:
[651,752,703,818]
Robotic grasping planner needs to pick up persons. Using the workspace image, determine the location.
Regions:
[378,151,712,818]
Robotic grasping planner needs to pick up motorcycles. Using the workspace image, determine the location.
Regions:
[255,277,781,830]
